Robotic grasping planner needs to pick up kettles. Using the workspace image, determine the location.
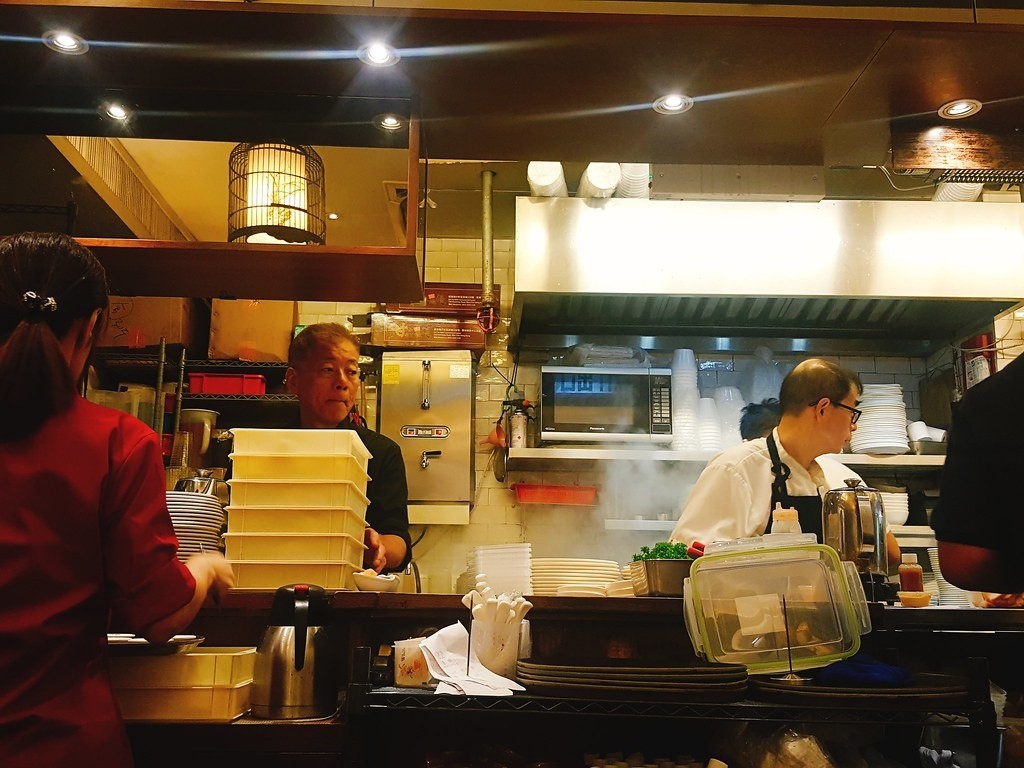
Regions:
[252,584,349,722]
[823,479,898,605]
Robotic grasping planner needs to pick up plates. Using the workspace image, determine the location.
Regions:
[849,383,908,452]
[928,547,972,608]
[516,664,748,695]
[165,491,223,564]
[619,164,650,198]
[466,543,534,596]
[531,558,635,597]
[932,181,984,202]
[577,161,621,197]
[528,161,567,197]
[755,672,976,707]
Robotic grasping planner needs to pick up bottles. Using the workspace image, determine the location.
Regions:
[772,502,804,534]
[897,553,924,593]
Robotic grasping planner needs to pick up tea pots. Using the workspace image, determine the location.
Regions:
[175,470,230,504]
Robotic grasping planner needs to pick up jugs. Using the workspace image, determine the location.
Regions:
[178,410,220,469]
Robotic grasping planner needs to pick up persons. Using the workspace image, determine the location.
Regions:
[0,232,235,768]
[207,322,413,574]
[930,351,1024,594]
[667,358,900,577]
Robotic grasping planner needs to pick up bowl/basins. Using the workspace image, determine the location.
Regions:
[672,348,699,447]
[716,384,753,448]
[354,572,399,591]
[882,492,910,525]
[699,399,721,452]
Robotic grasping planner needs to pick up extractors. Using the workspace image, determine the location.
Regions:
[510,200,1024,356]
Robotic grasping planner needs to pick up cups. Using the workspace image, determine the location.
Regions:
[166,430,193,469]
[470,621,519,682]
[394,641,429,686]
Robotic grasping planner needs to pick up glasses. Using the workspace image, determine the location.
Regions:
[808,400,862,424]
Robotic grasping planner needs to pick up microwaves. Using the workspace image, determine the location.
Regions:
[539,363,673,445]
